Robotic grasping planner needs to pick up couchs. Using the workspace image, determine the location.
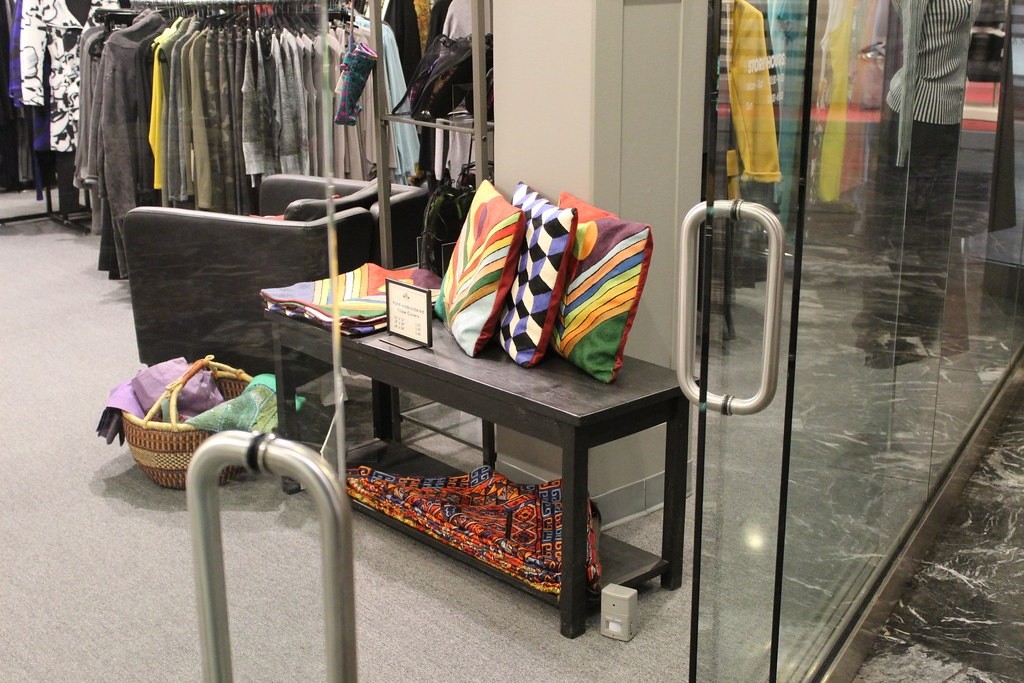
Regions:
[124,166,425,389]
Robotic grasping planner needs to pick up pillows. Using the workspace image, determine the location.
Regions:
[496,182,576,367]
[432,179,527,355]
[550,192,653,384]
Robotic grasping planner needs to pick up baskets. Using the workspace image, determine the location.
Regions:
[120,355,273,489]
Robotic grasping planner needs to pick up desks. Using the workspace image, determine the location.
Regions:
[263,266,698,640]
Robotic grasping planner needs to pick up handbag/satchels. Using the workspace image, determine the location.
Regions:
[417,161,494,280]
[391,33,493,122]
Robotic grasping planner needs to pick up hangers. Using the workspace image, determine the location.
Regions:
[131,0,357,36]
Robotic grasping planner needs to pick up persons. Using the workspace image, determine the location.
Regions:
[858,1,973,367]
